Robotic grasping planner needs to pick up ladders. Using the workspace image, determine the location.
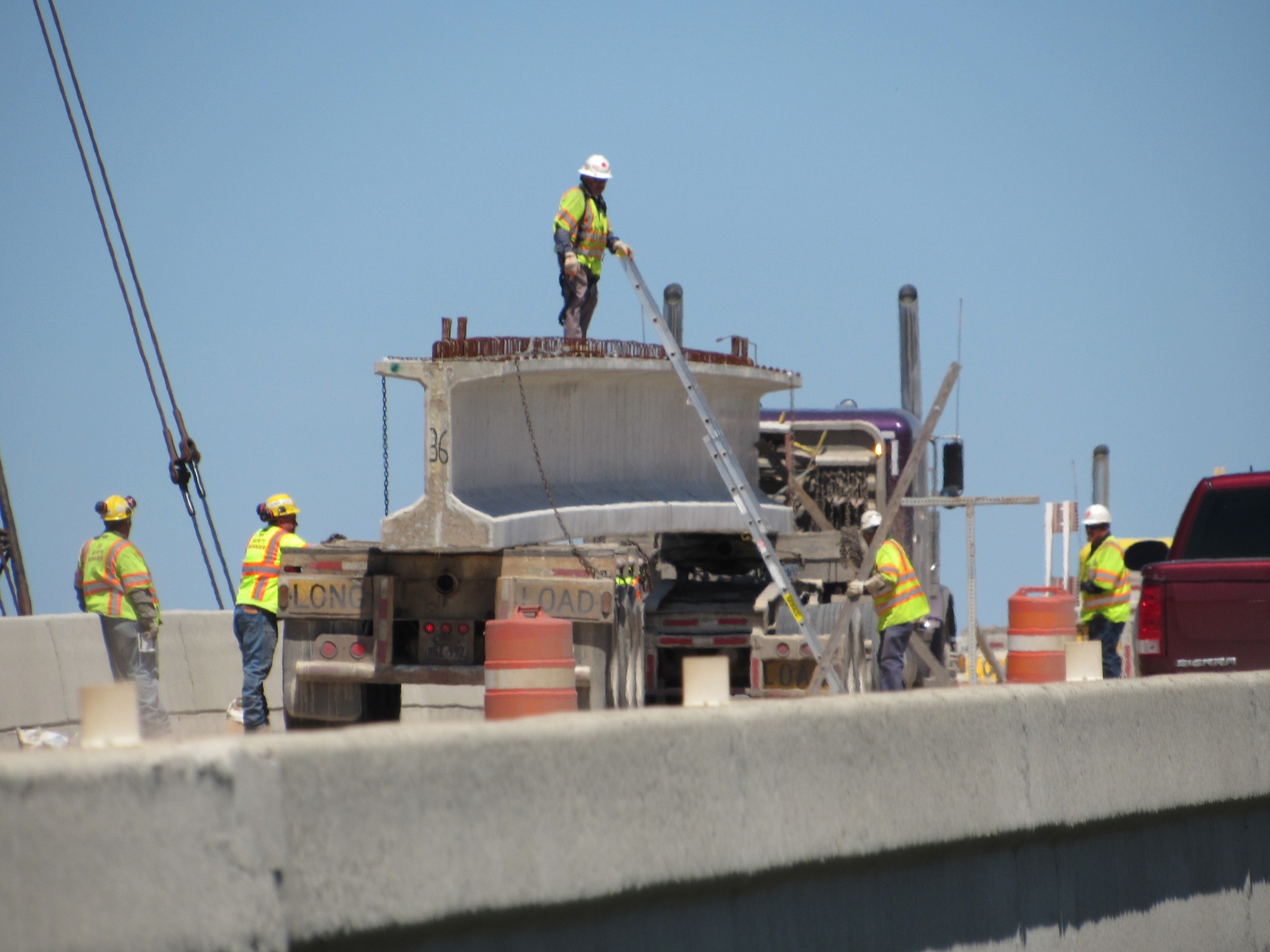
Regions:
[613,246,847,695]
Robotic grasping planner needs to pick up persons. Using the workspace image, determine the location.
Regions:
[75,495,176,745]
[553,154,633,345]
[1079,504,1131,679]
[846,510,930,692]
[234,493,311,735]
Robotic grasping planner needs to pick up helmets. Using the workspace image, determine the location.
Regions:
[101,496,134,522]
[265,495,300,517]
[1082,504,1112,526]
[579,154,613,179]
[858,510,883,532]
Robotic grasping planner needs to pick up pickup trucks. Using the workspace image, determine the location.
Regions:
[1123,469,1270,677]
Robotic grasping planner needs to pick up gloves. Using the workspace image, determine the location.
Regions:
[613,240,633,261]
[794,579,825,593]
[563,251,580,278]
[844,579,863,602]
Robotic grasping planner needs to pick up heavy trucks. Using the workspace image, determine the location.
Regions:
[277,408,967,737]
